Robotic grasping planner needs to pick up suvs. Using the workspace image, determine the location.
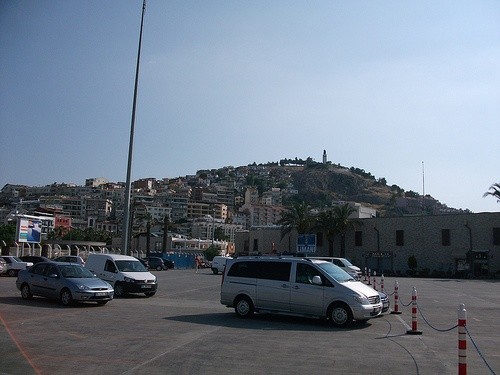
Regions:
[144,257,164,271]
[16,261,114,306]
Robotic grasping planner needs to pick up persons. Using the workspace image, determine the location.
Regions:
[194,254,200,272]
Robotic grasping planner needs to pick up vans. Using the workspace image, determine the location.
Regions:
[305,256,362,280]
[220,256,383,325]
[211,255,233,274]
[85,252,158,297]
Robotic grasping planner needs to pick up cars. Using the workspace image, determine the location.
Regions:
[0,258,7,274]
[376,289,390,314]
[18,256,53,264]
[0,255,33,276]
[163,257,174,269]
[52,255,85,266]
